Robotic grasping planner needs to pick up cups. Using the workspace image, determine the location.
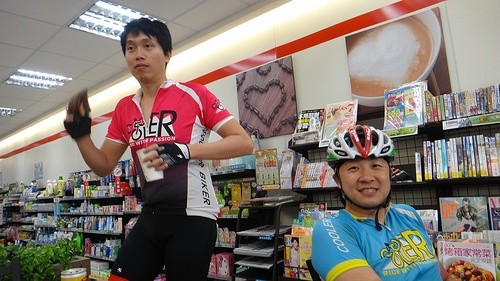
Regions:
[136,145,164,182]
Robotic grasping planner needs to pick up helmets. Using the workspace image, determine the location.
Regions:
[326,124,396,161]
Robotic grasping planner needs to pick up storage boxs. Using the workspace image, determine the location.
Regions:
[54,255,91,276]
[208,251,234,277]
[90,260,112,280]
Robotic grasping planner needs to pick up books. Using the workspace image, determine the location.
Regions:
[414,84,500,281]
[284,98,358,281]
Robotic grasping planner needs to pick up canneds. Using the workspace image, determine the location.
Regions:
[61,268,88,281]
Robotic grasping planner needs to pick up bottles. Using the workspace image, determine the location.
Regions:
[47,176,64,196]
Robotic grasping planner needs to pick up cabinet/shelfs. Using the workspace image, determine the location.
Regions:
[207,169,255,281]
[288,112,500,192]
[232,199,301,281]
[0,175,142,262]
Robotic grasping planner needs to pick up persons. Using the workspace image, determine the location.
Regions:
[64,17,254,281]
[457,199,479,232]
[311,125,455,281]
[290,237,299,272]
